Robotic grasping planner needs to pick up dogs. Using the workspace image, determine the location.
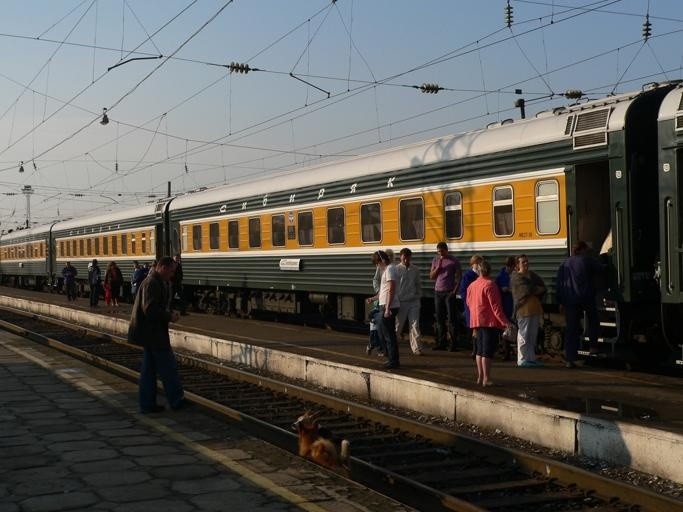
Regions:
[291,410,333,439]
[298,420,338,471]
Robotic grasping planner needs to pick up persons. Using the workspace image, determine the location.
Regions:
[367,242,608,388]
[127,256,195,413]
[61,253,187,316]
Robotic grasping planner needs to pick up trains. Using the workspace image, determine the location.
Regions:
[0,78,682,376]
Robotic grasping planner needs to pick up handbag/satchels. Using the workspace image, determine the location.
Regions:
[503,327,517,343]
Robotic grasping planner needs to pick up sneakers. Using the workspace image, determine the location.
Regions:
[431,344,456,352]
[142,405,164,414]
[522,360,544,368]
[565,360,576,368]
[415,350,423,356]
[377,349,400,369]
[172,398,192,409]
